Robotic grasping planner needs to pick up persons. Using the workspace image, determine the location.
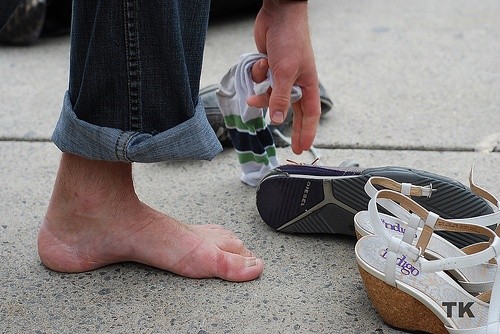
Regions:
[38,1,321,282]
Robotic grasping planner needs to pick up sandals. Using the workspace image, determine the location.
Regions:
[354,167,500,334]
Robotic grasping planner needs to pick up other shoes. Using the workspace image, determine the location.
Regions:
[194,68,335,144]
[255,163,498,250]
